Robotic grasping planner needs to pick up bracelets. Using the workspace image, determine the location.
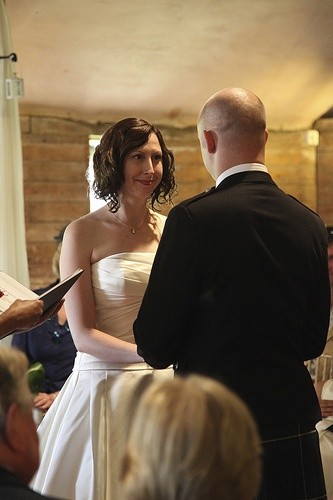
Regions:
[51,392,58,397]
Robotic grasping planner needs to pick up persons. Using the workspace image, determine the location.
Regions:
[11,225,79,427]
[133,86,332,500]
[28,118,175,500]
[110,374,261,499]
[0,299,70,500]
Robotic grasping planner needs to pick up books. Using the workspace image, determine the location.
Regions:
[0,268,85,315]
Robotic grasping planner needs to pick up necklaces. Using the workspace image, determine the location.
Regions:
[109,202,147,234]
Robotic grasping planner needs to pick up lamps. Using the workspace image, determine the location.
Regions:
[0,52,25,101]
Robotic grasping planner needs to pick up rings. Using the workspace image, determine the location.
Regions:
[46,314,54,321]
[44,398,47,401]
